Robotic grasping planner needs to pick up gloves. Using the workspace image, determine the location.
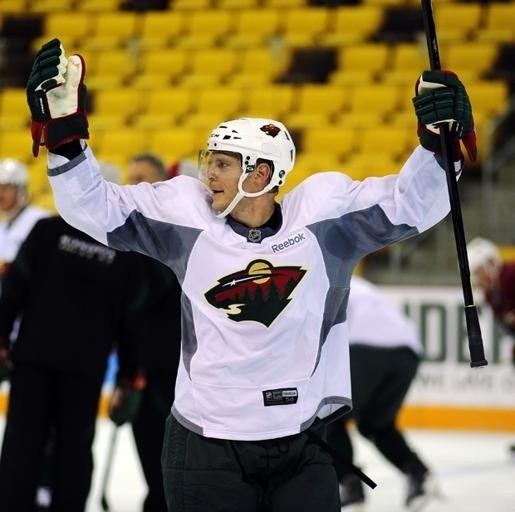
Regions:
[108,377,146,427]
[26,38,89,157]
[412,71,477,162]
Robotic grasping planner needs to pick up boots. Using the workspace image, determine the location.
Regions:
[341,482,366,508]
[402,452,428,505]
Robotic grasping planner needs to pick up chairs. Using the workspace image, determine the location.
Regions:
[1,1,515,218]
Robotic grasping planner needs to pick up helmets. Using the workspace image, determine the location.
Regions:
[198,118,296,186]
[466,238,496,272]
[0,159,30,187]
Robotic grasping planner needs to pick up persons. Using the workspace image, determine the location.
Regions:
[0,216,177,510]
[112,154,173,511]
[456,229,515,455]
[0,158,49,348]
[326,265,438,510]
[26,35,478,512]
